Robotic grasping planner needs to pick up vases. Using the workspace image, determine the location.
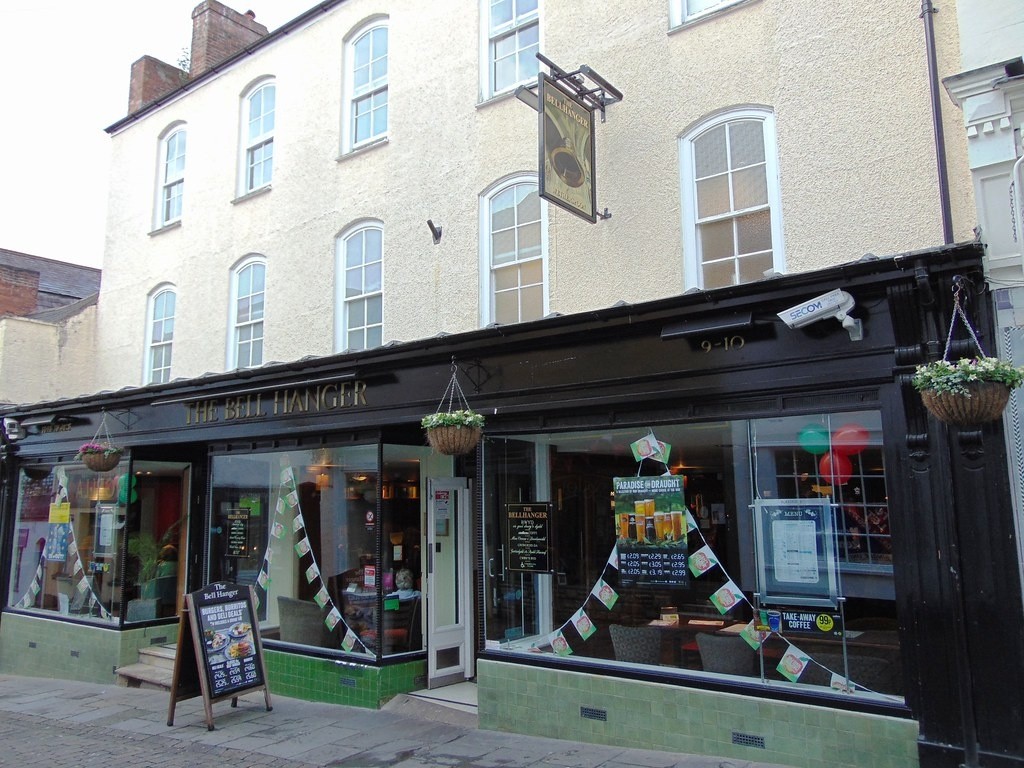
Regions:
[426,427,479,456]
[920,382,1012,438]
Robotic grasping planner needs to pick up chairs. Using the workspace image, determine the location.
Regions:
[697,631,753,673]
[276,595,343,649]
[126,576,176,619]
[362,596,421,655]
[609,623,682,667]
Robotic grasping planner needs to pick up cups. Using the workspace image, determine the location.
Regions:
[766,611,781,632]
[620,498,682,541]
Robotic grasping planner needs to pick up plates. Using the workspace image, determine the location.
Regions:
[206,633,230,651]
[225,640,254,660]
[228,621,251,637]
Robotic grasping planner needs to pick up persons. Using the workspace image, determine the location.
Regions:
[155,545,178,577]
[357,556,422,600]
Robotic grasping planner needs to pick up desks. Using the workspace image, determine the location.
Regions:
[647,618,897,655]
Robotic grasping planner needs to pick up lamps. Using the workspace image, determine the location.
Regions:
[407,482,418,500]
[382,481,392,499]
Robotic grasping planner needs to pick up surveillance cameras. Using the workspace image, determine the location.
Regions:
[777,288,856,330]
[3,418,19,433]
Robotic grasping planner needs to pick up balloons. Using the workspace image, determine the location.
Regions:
[799,421,870,486]
[114,473,138,505]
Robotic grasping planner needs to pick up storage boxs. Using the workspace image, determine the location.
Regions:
[660,614,679,620]
[661,607,677,614]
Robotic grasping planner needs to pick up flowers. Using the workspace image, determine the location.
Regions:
[73,443,123,462]
[82,453,119,472]
[901,357,1023,399]
[422,410,484,428]
